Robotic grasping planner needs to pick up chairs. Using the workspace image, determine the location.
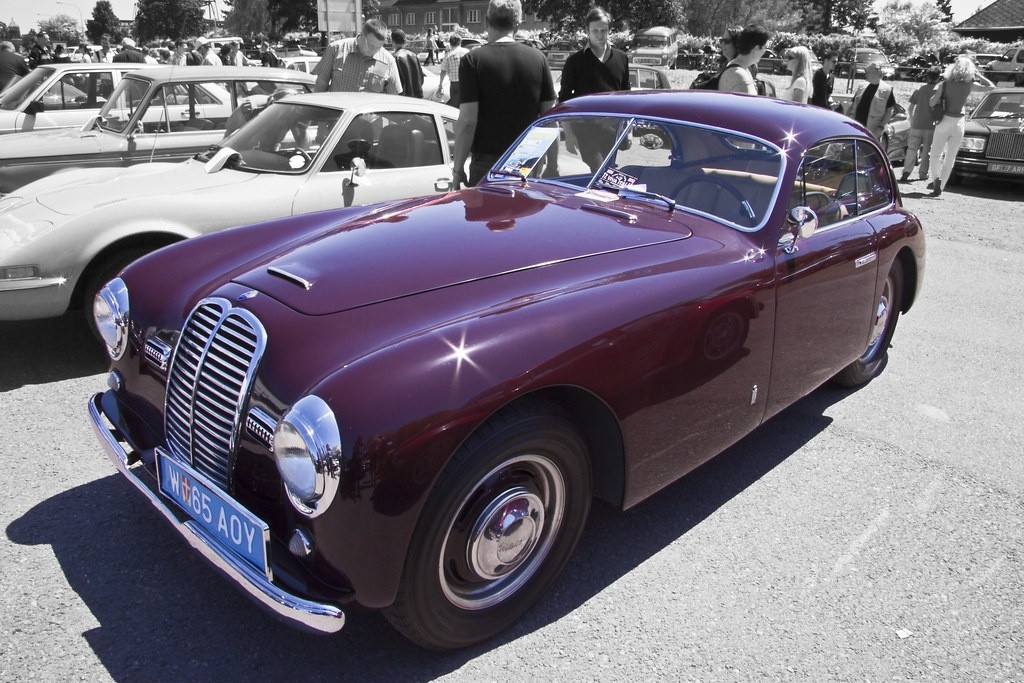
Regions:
[322,120,424,170]
[998,102,1019,111]
[180,119,216,131]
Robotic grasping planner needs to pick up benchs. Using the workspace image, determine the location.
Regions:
[611,164,836,240]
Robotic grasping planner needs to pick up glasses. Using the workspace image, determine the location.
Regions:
[363,36,382,51]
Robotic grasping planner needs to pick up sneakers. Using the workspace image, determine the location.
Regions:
[901,171,911,181]
[919,174,929,180]
[933,178,942,196]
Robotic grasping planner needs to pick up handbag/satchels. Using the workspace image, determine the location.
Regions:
[931,81,946,126]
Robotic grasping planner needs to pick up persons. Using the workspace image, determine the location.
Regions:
[223,87,311,153]
[438,35,471,109]
[810,51,841,110]
[314,19,404,145]
[261,42,278,68]
[845,62,896,143]
[453,0,561,191]
[558,8,632,174]
[901,56,997,196]
[711,24,771,97]
[424,25,441,66]
[0,32,257,99]
[786,46,812,104]
[389,28,423,99]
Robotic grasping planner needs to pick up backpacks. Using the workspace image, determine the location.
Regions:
[690,64,744,91]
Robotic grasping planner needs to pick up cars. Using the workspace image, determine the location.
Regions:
[1,26,679,198]
[820,46,1024,168]
[0,89,592,360]
[85,88,928,655]
[831,46,899,82]
[757,48,786,76]
[952,86,1024,183]
